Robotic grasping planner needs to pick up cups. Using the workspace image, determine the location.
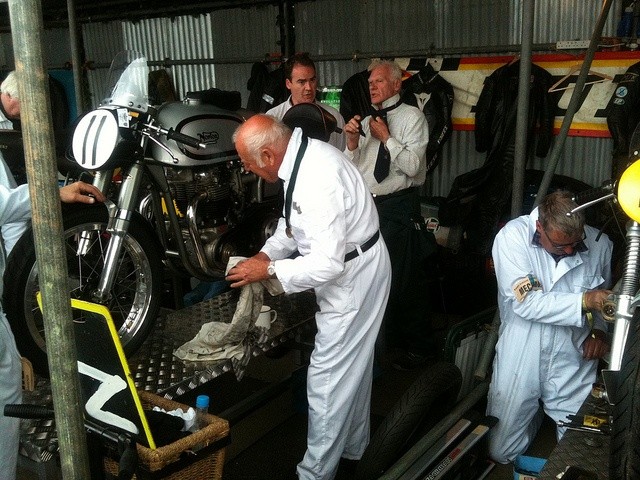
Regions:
[255,306,278,330]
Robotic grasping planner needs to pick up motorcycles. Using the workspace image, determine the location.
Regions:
[565,120,640,480]
[3,49,283,379]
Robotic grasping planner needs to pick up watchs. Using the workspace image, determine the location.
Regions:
[267,261,276,278]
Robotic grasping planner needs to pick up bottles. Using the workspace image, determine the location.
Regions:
[187,395,211,434]
[620,2,635,37]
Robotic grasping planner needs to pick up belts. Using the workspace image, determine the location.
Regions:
[344,231,379,262]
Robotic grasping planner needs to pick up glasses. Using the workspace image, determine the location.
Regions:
[543,227,587,249]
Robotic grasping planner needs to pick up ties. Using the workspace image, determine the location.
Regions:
[357,98,404,184]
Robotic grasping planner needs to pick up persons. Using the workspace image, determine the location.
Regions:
[225,114,392,479]
[0,182,107,479]
[482,188,615,466]
[0,71,30,260]
[263,56,346,152]
[343,57,434,373]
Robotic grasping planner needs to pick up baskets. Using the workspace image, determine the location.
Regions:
[102,389,231,480]
